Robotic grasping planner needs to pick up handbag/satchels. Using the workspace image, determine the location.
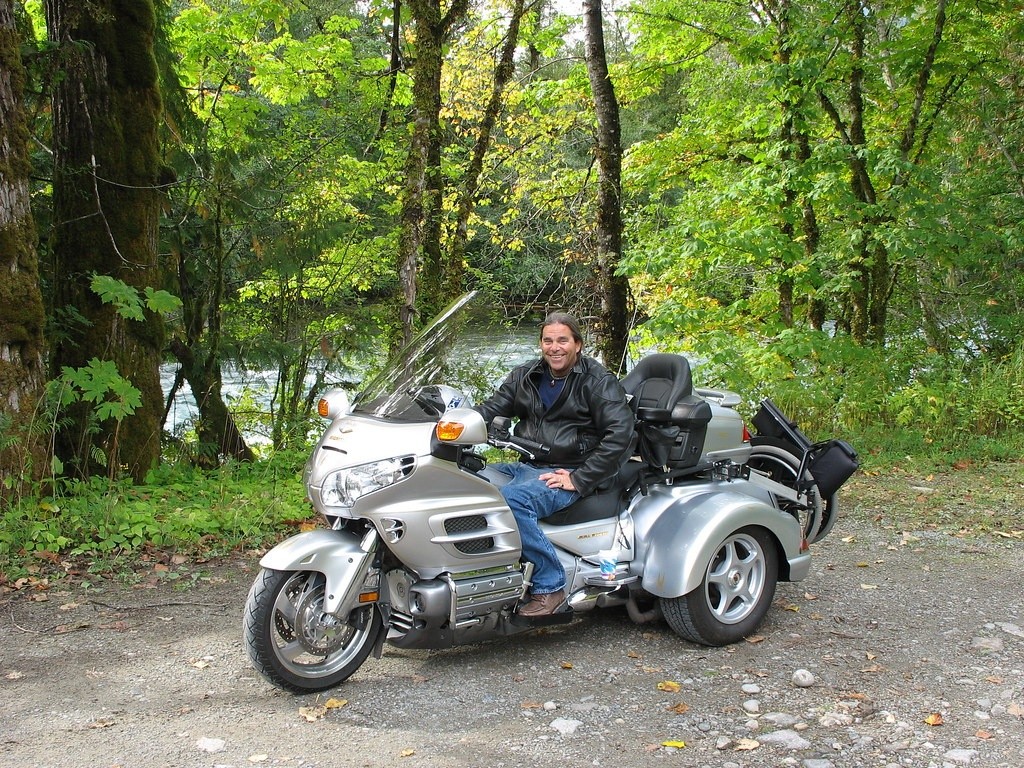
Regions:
[795,439,859,500]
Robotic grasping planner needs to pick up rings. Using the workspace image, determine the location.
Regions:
[559,479,561,483]
[559,483,564,488]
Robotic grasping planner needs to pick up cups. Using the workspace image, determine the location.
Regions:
[599,549,618,580]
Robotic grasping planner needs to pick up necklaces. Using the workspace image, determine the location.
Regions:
[548,366,567,387]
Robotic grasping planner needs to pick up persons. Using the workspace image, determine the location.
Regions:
[472,312,638,616]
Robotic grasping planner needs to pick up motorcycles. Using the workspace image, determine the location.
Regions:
[242,291,858,694]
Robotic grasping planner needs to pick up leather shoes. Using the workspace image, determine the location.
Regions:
[519,588,568,616]
